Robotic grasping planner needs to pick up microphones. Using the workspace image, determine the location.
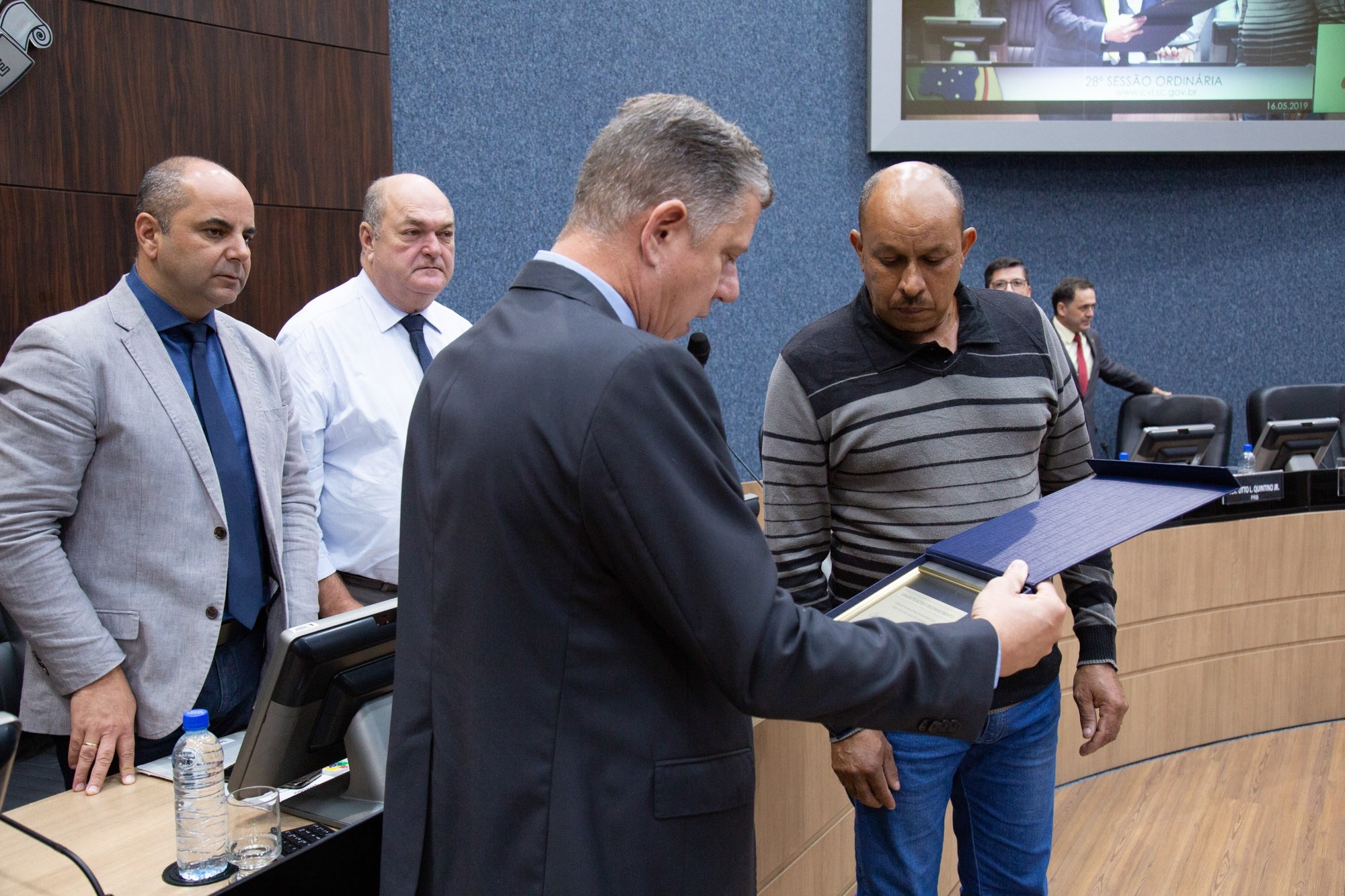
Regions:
[687,332,764,488]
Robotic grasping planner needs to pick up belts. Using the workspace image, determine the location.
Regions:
[217,622,243,645]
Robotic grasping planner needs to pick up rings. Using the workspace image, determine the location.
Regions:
[82,741,98,747]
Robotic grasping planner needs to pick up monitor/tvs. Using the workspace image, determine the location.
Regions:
[1252,417,1341,473]
[1131,423,1216,466]
[228,595,403,828]
[865,0,1345,153]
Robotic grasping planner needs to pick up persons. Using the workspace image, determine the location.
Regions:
[1234,0,1345,122]
[761,161,1130,896]
[1032,0,1194,121]
[375,92,1071,896]
[1049,278,1172,461]
[272,174,474,621]
[0,155,321,796]
[983,257,1032,299]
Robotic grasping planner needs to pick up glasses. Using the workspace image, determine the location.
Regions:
[990,278,1026,291]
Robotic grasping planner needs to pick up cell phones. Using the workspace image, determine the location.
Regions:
[224,764,322,789]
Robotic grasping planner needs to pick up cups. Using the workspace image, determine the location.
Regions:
[227,786,282,871]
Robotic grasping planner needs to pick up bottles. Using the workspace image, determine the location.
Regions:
[171,709,228,881]
[1119,452,1129,460]
[1238,443,1255,475]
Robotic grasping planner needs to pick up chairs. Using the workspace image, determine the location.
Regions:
[1114,393,1234,466]
[1247,381,1345,470]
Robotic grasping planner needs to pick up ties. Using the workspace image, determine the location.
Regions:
[400,315,433,375]
[180,323,264,630]
[1073,332,1088,397]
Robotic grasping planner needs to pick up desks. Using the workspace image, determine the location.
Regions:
[900,60,1318,121]
